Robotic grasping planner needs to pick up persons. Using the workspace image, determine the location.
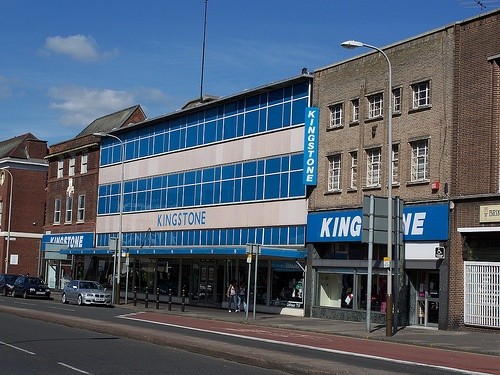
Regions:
[226,279,239,312]
[237,279,247,312]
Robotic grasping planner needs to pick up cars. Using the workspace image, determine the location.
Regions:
[61,280,112,306]
[0,273,24,296]
[12,276,51,299]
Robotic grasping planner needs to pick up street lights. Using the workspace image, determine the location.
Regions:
[341,40,393,337]
[94,132,125,304]
